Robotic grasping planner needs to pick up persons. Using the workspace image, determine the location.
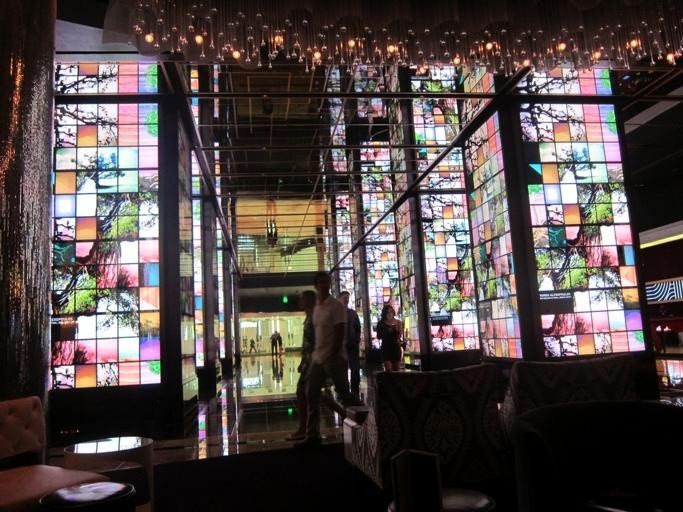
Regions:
[275,331,284,355]
[283,290,347,442]
[248,338,258,353]
[290,273,352,451]
[376,303,404,370]
[335,290,360,406]
[271,358,284,383]
[270,332,280,356]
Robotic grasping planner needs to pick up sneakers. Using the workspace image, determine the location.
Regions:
[293,437,322,447]
[284,433,305,441]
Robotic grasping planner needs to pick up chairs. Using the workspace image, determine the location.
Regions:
[510,399,659,511]
[0,381,176,511]
[495,348,660,442]
[344,363,506,503]
[419,348,486,373]
